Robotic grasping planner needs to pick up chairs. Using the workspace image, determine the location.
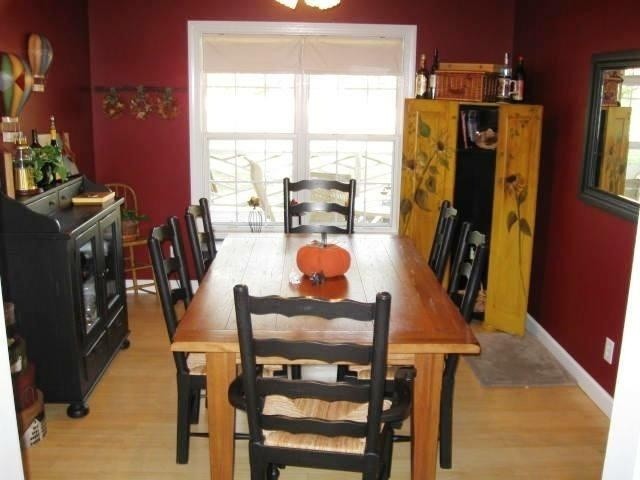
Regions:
[185,198,216,285]
[283,177,356,233]
[228,284,416,480]
[148,216,286,479]
[103,183,162,306]
[426,200,458,284]
[336,219,489,469]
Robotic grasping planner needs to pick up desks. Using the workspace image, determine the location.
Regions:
[169,232,481,480]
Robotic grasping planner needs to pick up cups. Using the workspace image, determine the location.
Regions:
[497,78,517,98]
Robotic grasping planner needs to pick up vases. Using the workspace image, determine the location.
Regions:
[249,210,263,232]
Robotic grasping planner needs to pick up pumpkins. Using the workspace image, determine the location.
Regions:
[297,241,350,278]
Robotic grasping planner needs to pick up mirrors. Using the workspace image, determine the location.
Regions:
[576,48,640,225]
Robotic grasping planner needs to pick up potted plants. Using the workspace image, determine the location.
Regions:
[114,196,149,242]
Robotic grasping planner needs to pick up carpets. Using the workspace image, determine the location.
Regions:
[461,332,579,389]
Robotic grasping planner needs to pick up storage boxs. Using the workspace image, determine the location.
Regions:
[4,303,48,449]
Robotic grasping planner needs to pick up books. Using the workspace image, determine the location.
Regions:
[72,191,115,205]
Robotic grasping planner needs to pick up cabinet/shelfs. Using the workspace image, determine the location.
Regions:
[0,169,131,419]
[594,106,632,196]
[397,98,544,338]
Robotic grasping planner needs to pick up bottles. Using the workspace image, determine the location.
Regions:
[0,115,42,197]
[414,48,439,98]
[49,115,58,145]
[501,52,525,103]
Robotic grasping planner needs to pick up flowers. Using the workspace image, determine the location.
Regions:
[248,196,261,210]
[291,198,302,225]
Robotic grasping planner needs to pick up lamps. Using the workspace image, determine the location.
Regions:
[274,0,341,11]
